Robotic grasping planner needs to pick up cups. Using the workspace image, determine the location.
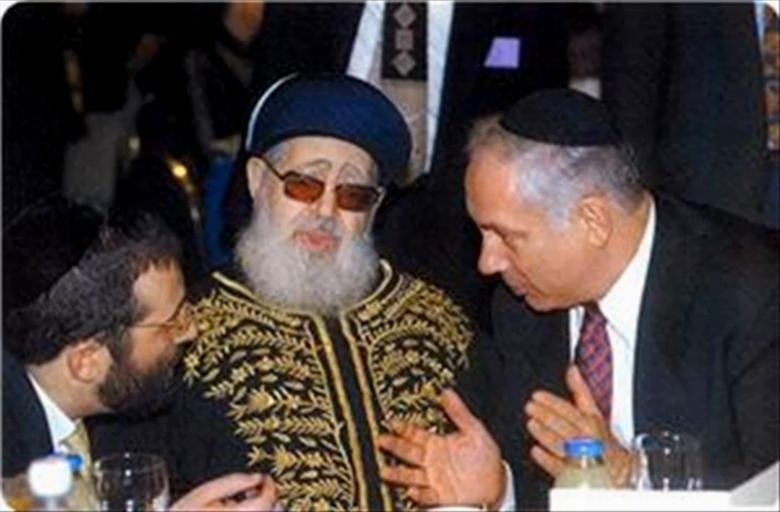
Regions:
[91,453,171,511]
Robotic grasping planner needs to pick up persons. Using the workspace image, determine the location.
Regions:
[0,210,282,512]
[6,0,766,272]
[375,84,780,512]
[173,71,486,512]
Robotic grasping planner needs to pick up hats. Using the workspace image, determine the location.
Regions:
[4,200,103,313]
[493,86,630,152]
[242,65,416,182]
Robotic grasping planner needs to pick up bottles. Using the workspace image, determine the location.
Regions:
[16,450,91,511]
[552,436,612,489]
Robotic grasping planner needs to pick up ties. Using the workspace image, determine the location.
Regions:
[380,0,428,191]
[575,302,615,439]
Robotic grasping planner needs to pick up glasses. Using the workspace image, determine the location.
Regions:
[279,169,386,215]
[140,293,194,339]
